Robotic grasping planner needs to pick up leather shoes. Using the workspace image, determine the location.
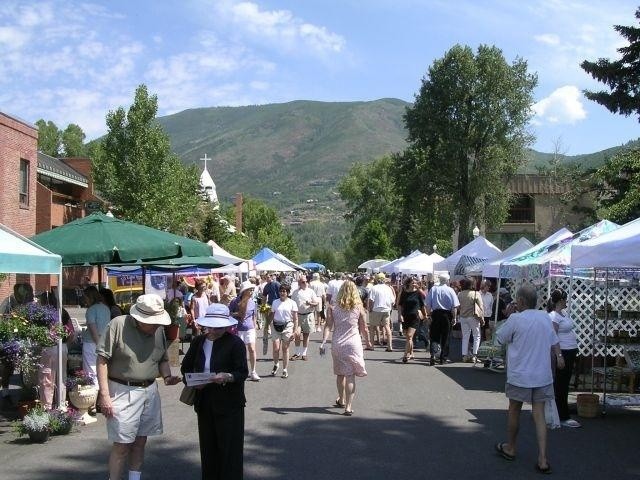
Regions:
[440,359,449,364]
[429,357,436,366]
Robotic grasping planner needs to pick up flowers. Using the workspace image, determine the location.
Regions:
[65,370,96,389]
[48,402,83,427]
[0,302,72,370]
[12,400,61,437]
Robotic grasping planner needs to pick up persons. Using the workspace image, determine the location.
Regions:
[547,287,582,428]
[1,269,508,414]
[95,294,182,479]
[180,303,248,480]
[497,286,559,475]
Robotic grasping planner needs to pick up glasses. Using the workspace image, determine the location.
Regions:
[302,281,308,283]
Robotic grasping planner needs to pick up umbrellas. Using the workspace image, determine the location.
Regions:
[27,210,213,291]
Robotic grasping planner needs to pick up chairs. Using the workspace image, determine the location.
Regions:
[472,320,509,373]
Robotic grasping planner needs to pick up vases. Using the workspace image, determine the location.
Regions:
[69,384,99,425]
[57,424,70,434]
[28,431,50,442]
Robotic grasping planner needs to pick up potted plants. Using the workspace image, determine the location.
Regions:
[166,302,179,340]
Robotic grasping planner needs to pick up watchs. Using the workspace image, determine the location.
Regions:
[557,353,563,357]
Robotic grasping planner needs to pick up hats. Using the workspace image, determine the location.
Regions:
[195,303,239,328]
[239,281,255,294]
[129,294,172,326]
[14,283,34,303]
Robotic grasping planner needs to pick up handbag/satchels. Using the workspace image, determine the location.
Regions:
[273,320,291,332]
[180,385,197,406]
[473,291,484,321]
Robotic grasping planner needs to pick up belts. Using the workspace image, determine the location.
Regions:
[298,312,312,315]
[108,376,154,387]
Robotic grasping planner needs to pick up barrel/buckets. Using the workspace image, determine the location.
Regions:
[577,394,599,418]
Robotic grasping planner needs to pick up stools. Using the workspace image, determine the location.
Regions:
[594,367,635,394]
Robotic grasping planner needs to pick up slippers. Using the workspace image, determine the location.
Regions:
[344,410,353,416]
[538,462,552,474]
[336,398,346,408]
[494,442,515,460]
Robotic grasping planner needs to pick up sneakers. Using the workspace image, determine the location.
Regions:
[560,419,582,428]
[282,372,288,378]
[290,353,301,361]
[302,355,308,360]
[462,356,471,362]
[472,355,482,362]
[251,374,260,381]
[271,365,279,375]
